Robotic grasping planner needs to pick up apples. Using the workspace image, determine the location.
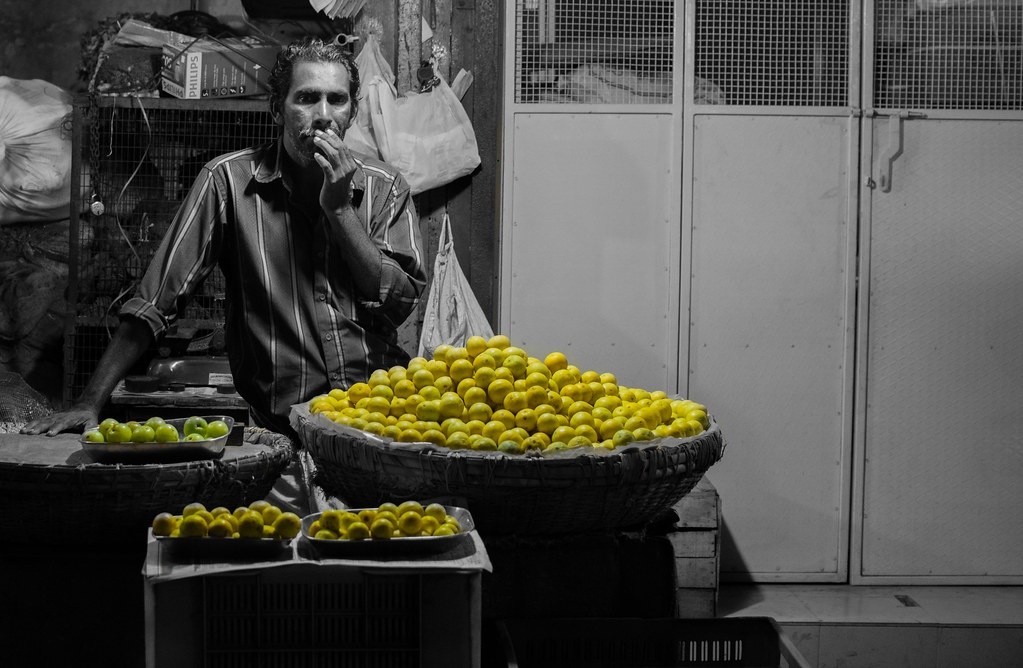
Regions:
[81,415,230,445]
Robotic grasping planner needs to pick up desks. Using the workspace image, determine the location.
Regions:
[140,506,494,668]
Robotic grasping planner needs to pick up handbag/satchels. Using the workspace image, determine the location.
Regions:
[343,32,397,161]
[417,213,496,360]
[369,67,482,196]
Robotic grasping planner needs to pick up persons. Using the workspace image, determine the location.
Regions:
[20,41,431,459]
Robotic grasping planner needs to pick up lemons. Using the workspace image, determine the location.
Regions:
[309,501,460,540]
[308,335,708,455]
[152,499,302,539]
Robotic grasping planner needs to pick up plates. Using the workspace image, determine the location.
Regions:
[301,505,474,556]
[78,416,234,461]
[152,515,292,555]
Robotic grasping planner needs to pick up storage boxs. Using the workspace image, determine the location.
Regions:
[162,34,282,100]
[666,474,721,618]
[497,617,811,668]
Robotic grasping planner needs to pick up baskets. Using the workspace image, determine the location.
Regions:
[0,411,283,515]
[292,388,725,534]
